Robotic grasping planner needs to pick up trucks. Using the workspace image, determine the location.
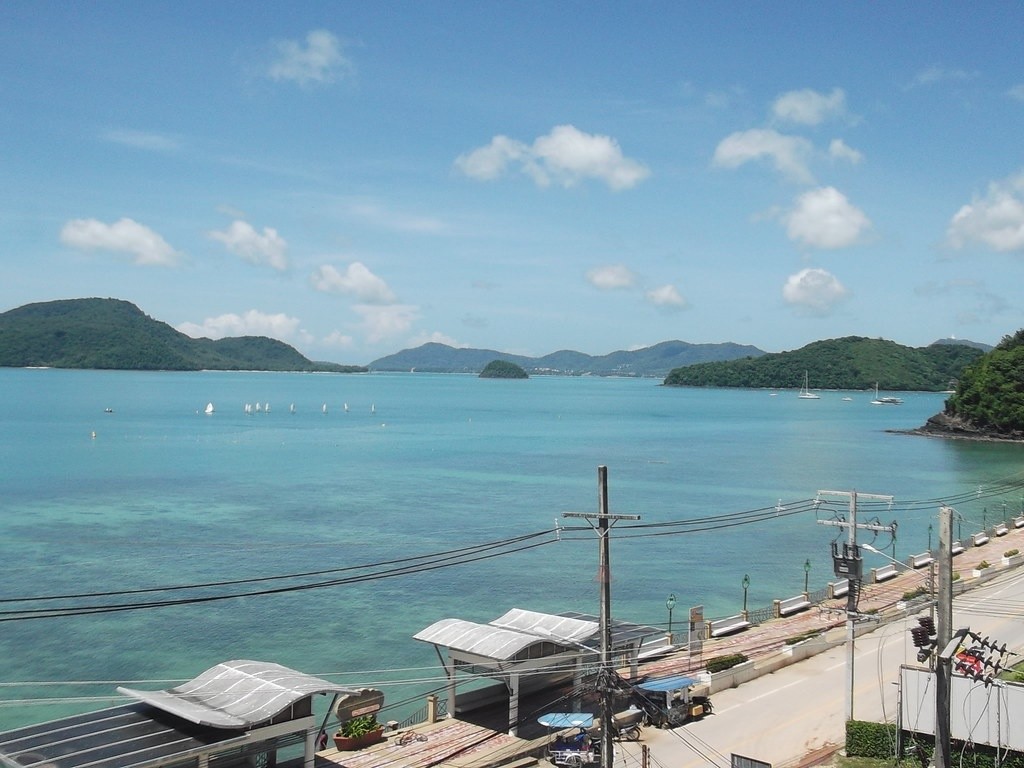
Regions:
[537,712,606,768]
[635,675,711,730]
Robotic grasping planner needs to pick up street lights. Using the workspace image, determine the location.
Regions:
[804,558,811,591]
[665,593,677,633]
[891,496,1024,560]
[741,573,751,610]
[859,545,934,670]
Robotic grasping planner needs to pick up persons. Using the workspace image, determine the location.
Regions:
[318,730,328,751]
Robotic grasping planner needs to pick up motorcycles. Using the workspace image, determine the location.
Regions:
[597,719,643,740]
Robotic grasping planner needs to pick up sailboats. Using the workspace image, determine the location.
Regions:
[871,380,903,406]
[798,370,821,399]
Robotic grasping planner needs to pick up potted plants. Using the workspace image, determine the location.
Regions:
[332,713,386,751]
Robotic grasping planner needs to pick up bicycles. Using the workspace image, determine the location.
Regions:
[393,730,429,745]
